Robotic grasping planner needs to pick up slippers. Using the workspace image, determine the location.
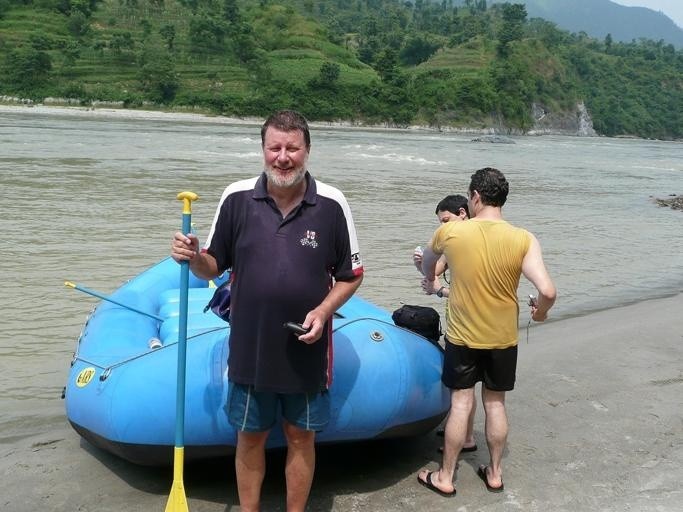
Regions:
[480,464,504,492]
[437,428,445,435]
[418,471,456,497]
[438,444,477,453]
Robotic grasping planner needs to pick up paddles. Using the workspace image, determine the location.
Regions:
[165,192,198,512]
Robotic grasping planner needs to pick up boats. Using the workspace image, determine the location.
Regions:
[61,248,452,472]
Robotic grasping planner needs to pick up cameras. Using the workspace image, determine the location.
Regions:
[284,321,306,336]
[527,295,537,309]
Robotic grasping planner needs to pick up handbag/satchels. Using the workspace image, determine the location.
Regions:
[391,306,442,342]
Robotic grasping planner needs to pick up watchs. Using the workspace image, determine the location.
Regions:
[435,286,444,298]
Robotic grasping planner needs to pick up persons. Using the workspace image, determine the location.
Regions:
[413,195,469,297]
[171,110,363,512]
[417,168,556,497]
